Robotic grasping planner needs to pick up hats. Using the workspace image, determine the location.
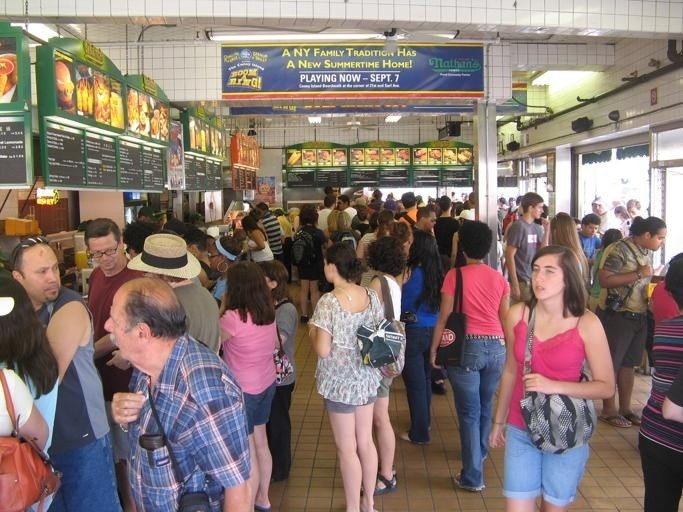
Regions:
[127,234,201,279]
[352,198,369,210]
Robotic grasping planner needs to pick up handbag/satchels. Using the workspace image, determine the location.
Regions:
[520,371,597,455]
[426,312,466,366]
[0,434,61,511]
[273,349,294,384]
[357,319,406,378]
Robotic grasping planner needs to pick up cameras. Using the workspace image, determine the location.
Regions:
[606,295,623,312]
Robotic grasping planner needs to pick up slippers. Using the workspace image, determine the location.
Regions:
[598,414,642,427]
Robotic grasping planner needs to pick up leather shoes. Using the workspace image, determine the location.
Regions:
[399,426,431,445]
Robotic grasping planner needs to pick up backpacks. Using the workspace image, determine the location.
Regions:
[337,230,357,251]
[290,228,320,266]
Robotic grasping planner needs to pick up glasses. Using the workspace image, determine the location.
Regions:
[86,241,118,259]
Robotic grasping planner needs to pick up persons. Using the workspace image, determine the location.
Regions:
[638,253,682,512]
[489,245,614,512]
[496,191,667,428]
[306,243,384,512]
[428,219,511,492]
[1,201,298,512]
[360,237,406,496]
[294,187,478,445]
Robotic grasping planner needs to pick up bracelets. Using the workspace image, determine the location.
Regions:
[491,416,504,425]
[119,424,129,433]
[637,271,641,279]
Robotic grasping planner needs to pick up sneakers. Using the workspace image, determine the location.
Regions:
[454,473,486,491]
[431,381,446,394]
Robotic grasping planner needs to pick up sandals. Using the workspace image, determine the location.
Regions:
[361,462,397,496]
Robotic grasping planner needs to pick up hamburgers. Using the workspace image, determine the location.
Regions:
[55,60,75,108]
